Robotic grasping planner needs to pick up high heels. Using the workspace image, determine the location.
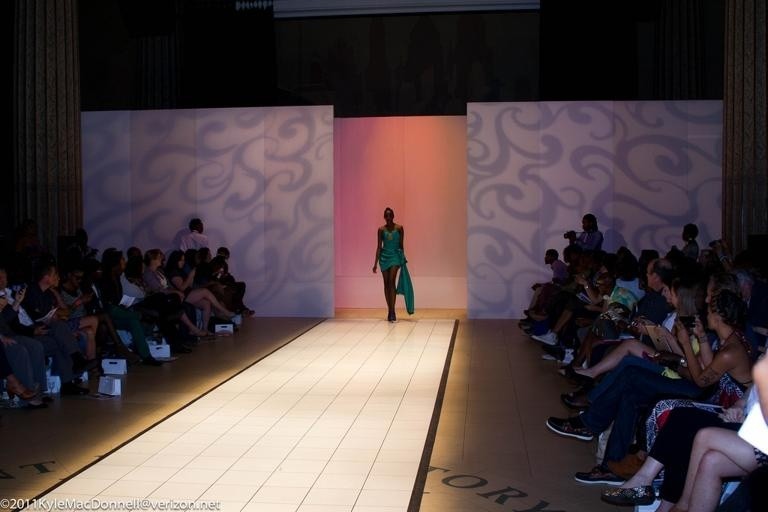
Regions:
[388,311,396,323]
[565,364,593,392]
[7,380,36,400]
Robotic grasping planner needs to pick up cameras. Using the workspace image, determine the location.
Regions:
[564,231,576,239]
[709,240,721,247]
[676,316,696,328]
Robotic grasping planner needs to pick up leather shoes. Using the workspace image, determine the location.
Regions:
[518,310,544,335]
[143,355,162,365]
[28,394,54,409]
[560,394,590,411]
[60,388,89,394]
[176,345,193,353]
[600,487,654,506]
[607,454,644,480]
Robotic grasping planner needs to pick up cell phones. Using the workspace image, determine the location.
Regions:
[19,282,28,295]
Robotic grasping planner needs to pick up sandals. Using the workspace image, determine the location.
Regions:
[188,329,215,337]
[224,310,236,318]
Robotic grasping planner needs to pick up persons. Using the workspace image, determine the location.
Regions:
[373,209,414,319]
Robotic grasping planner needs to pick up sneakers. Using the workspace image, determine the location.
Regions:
[573,464,625,486]
[530,329,557,346]
[541,348,574,367]
[545,415,593,441]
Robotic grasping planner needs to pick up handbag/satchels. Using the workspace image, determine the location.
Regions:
[649,351,684,372]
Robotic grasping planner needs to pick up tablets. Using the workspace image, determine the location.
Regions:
[646,324,672,353]
